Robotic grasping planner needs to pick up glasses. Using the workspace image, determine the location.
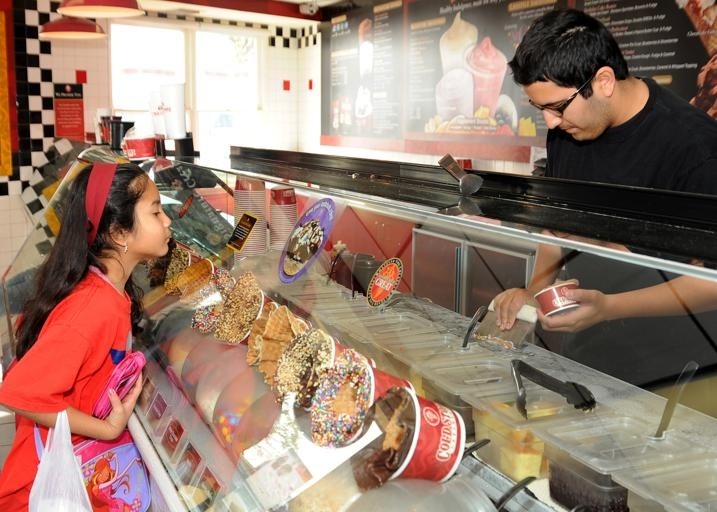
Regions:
[528,76,592,118]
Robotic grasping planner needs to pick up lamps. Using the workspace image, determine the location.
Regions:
[56,0,144,19]
[39,15,106,41]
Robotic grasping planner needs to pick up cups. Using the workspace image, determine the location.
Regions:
[464,44,508,119]
[440,25,479,75]
[435,71,473,123]
[156,82,187,142]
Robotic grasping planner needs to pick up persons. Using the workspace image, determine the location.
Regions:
[492,9,717,411]
[1,162,172,512]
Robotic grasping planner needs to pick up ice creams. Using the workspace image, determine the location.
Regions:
[284,221,323,276]
[435,11,507,121]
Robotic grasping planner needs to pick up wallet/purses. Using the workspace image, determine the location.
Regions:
[91,350,147,422]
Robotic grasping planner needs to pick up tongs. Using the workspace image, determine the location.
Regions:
[507,357,600,422]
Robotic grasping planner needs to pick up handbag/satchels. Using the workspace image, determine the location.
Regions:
[72,424,152,512]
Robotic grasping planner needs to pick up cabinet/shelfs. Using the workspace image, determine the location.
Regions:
[98,154,717,512]
